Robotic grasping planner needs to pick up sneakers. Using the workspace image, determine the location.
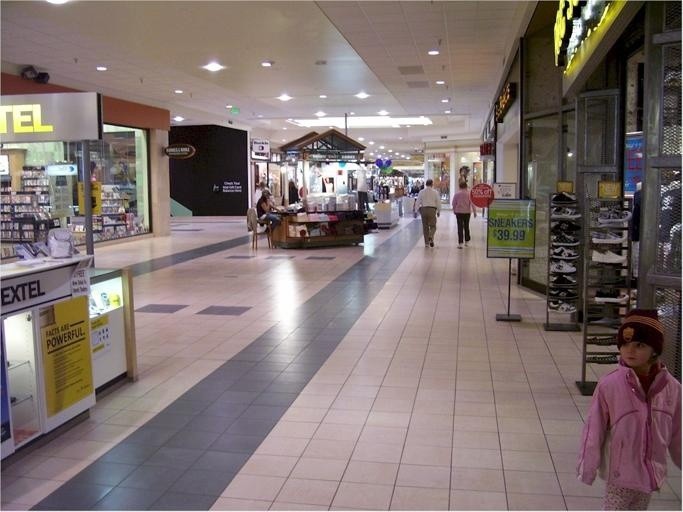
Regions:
[591,206,631,264]
[457,242,472,249]
[425,243,433,247]
[594,289,630,303]
[548,193,582,315]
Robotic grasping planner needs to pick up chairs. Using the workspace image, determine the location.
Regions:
[251,207,274,250]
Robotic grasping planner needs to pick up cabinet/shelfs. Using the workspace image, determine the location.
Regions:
[575,196,638,397]
[543,191,582,332]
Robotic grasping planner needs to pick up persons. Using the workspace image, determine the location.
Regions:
[452,182,476,249]
[282,182,298,206]
[257,190,281,233]
[577,309,681,511]
[414,179,440,248]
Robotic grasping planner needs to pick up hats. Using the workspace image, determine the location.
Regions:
[262,190,271,196]
[617,309,664,356]
